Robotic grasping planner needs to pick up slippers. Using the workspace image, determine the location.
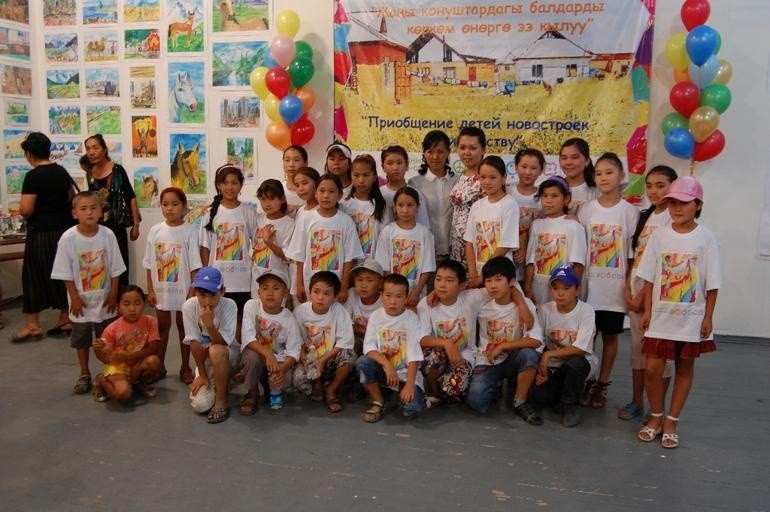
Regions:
[206,406,230,424]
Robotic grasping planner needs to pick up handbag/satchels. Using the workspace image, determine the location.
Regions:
[107,190,141,228]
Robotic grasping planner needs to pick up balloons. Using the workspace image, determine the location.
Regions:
[249,9,316,152]
[660,0,733,162]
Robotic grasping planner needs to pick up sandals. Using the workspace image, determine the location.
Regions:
[422,392,464,410]
[92,379,109,402]
[7,325,43,342]
[308,378,344,412]
[579,377,612,408]
[46,320,72,337]
[133,380,157,398]
[239,391,259,414]
[363,400,387,423]
[617,401,680,448]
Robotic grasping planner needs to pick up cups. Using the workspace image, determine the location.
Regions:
[0,217,12,234]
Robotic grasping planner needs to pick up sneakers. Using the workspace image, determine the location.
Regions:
[270,393,286,410]
[179,365,194,384]
[514,401,543,425]
[147,367,167,384]
[563,401,580,427]
[72,374,90,394]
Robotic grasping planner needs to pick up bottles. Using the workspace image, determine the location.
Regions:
[306,345,319,380]
[268,375,283,410]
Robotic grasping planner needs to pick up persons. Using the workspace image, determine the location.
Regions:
[50,191,128,392]
[182,264,238,424]
[91,285,162,406]
[639,176,722,448]
[80,133,139,301]
[240,128,597,422]
[9,132,75,344]
[576,151,637,410]
[199,163,259,344]
[616,165,677,424]
[143,188,197,383]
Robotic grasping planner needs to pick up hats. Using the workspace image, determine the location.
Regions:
[659,177,703,203]
[549,263,581,287]
[256,270,291,290]
[325,144,352,160]
[192,266,224,293]
[349,255,385,278]
